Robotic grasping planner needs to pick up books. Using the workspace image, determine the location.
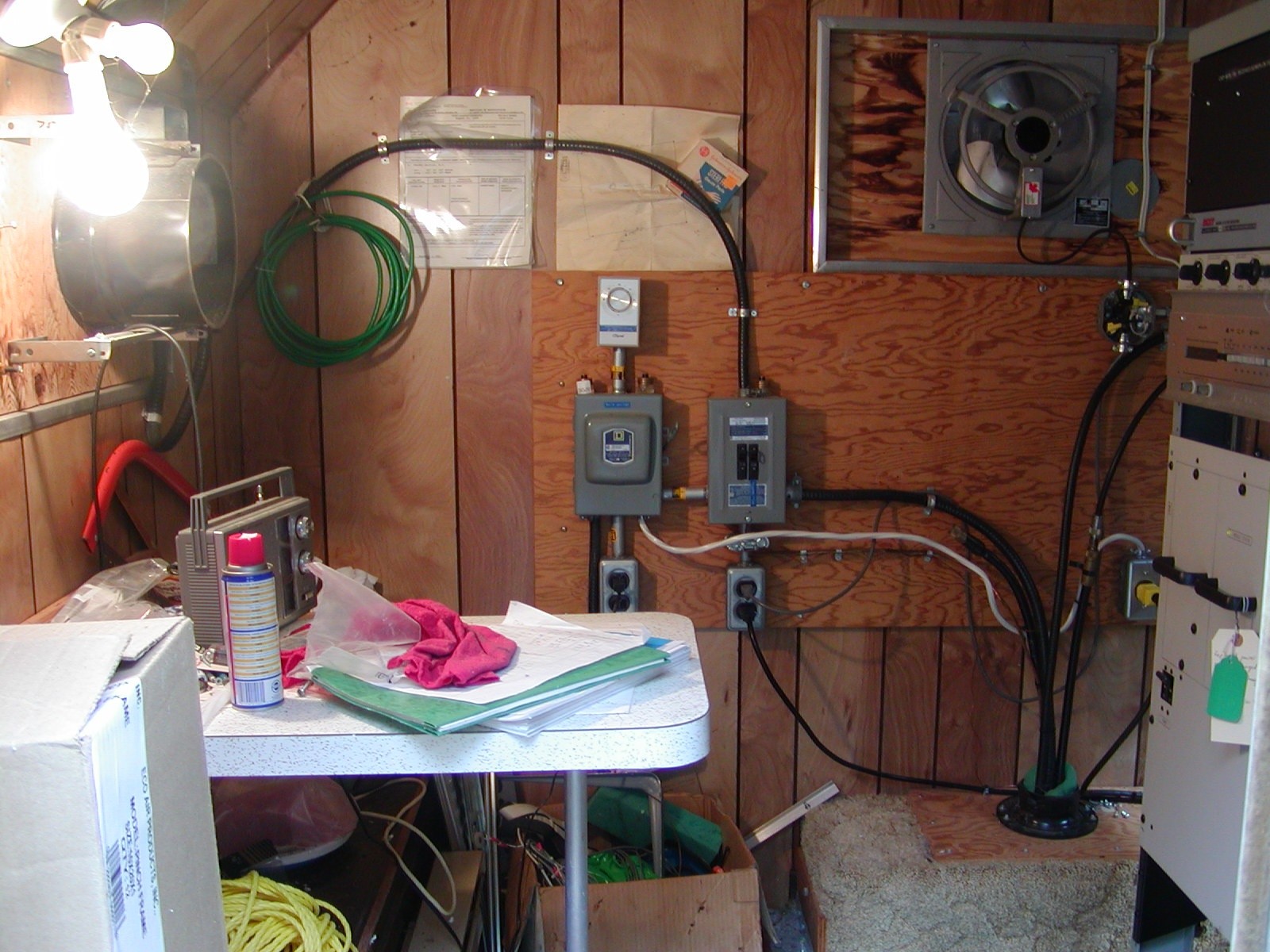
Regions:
[307,643,670,735]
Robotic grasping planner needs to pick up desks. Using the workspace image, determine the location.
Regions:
[207,612,715,951]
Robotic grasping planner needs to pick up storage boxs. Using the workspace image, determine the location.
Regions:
[0,616,228,952]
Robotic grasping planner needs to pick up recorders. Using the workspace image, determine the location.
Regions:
[175,467,318,648]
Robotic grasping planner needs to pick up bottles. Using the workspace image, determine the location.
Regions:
[218,533,284,711]
